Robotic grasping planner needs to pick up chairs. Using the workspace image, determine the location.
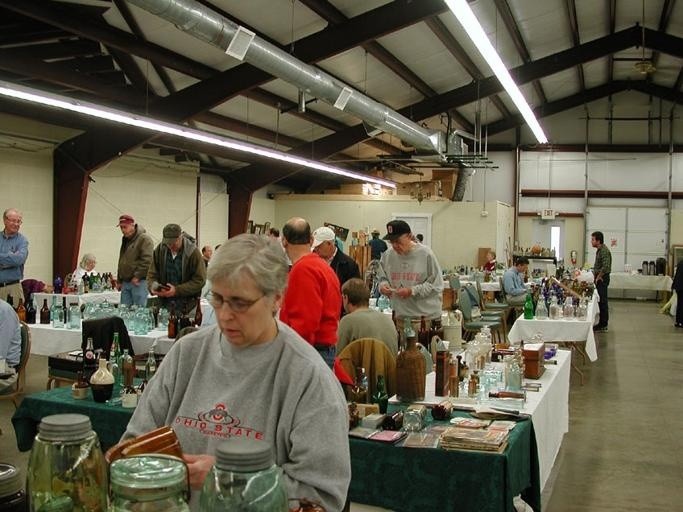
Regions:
[330,264,527,392]
[0,318,32,412]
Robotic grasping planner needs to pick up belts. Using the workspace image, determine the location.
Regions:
[0,279,20,287]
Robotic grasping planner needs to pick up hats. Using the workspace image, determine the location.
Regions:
[163,224,181,244]
[310,227,335,252]
[383,221,411,240]
[117,215,134,227]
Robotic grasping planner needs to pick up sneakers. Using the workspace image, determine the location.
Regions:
[593,323,608,333]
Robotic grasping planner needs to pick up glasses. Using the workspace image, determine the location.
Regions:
[204,289,265,313]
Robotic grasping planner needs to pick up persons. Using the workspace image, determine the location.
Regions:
[590,231,611,332]
[113,214,153,309]
[117,231,351,512]
[278,216,341,376]
[501,257,537,306]
[0,206,28,309]
[482,251,496,270]
[672,259,682,328]
[336,277,397,364]
[0,297,21,400]
[145,218,443,338]
[66,251,100,290]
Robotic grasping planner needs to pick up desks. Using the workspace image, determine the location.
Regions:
[579,267,669,301]
[20,285,218,360]
[11,349,575,512]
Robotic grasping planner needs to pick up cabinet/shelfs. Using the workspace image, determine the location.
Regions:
[510,271,600,387]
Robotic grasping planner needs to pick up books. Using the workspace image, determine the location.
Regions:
[439,426,510,455]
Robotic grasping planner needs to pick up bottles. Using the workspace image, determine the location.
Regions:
[24,411,109,512]
[70,332,159,407]
[6,293,192,339]
[0,463,28,512]
[521,272,588,323]
[198,436,290,512]
[106,454,188,511]
[53,271,115,295]
[342,326,543,431]
